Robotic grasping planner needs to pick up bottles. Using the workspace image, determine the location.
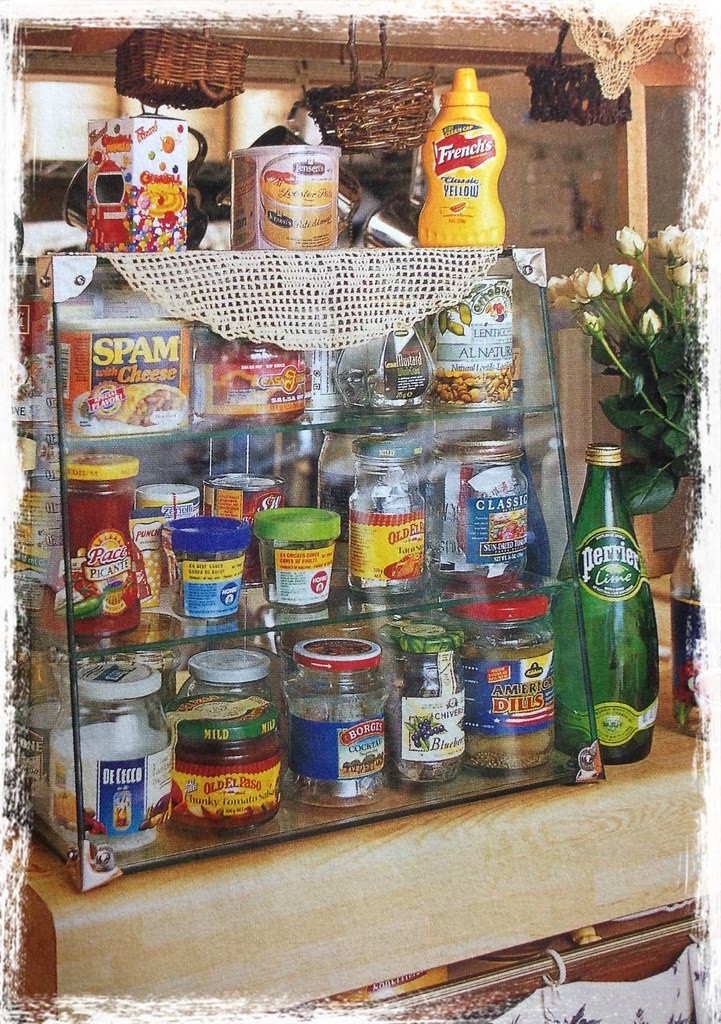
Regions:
[552,441,659,766]
[418,68,506,248]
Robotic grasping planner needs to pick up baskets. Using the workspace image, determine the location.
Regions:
[524,20,632,127]
[114,28,248,110]
[304,16,437,163]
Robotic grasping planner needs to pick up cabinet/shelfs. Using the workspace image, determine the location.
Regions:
[16,248,607,894]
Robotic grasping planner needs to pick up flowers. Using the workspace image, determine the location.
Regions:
[546,224,705,516]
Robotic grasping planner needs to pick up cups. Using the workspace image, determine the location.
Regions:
[164,508,341,619]
[229,145,341,250]
